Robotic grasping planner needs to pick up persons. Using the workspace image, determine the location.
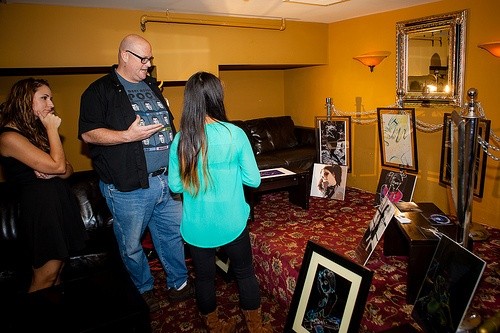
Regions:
[169,72,275,333]
[0,78,77,293]
[79,34,198,302]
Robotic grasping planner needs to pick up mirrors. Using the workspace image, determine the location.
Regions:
[395,8,464,109]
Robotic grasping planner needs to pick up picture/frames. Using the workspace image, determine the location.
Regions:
[282,240,374,333]
[438,112,491,198]
[377,107,418,172]
[314,116,353,174]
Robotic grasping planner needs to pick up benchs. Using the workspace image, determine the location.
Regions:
[244,166,310,222]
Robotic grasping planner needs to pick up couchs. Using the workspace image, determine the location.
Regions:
[0,170,129,333]
[230,115,319,183]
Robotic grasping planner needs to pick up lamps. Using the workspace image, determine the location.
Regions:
[352,55,387,73]
[477,42,500,58]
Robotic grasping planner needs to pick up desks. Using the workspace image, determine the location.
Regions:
[382,202,473,305]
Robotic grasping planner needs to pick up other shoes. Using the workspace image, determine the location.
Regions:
[168,282,195,301]
[141,289,160,312]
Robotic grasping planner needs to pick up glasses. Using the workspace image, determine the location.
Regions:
[125,50,154,64]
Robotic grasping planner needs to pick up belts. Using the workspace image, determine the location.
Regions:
[148,169,165,178]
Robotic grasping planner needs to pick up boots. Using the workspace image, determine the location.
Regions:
[199,306,236,333]
[241,306,273,333]
